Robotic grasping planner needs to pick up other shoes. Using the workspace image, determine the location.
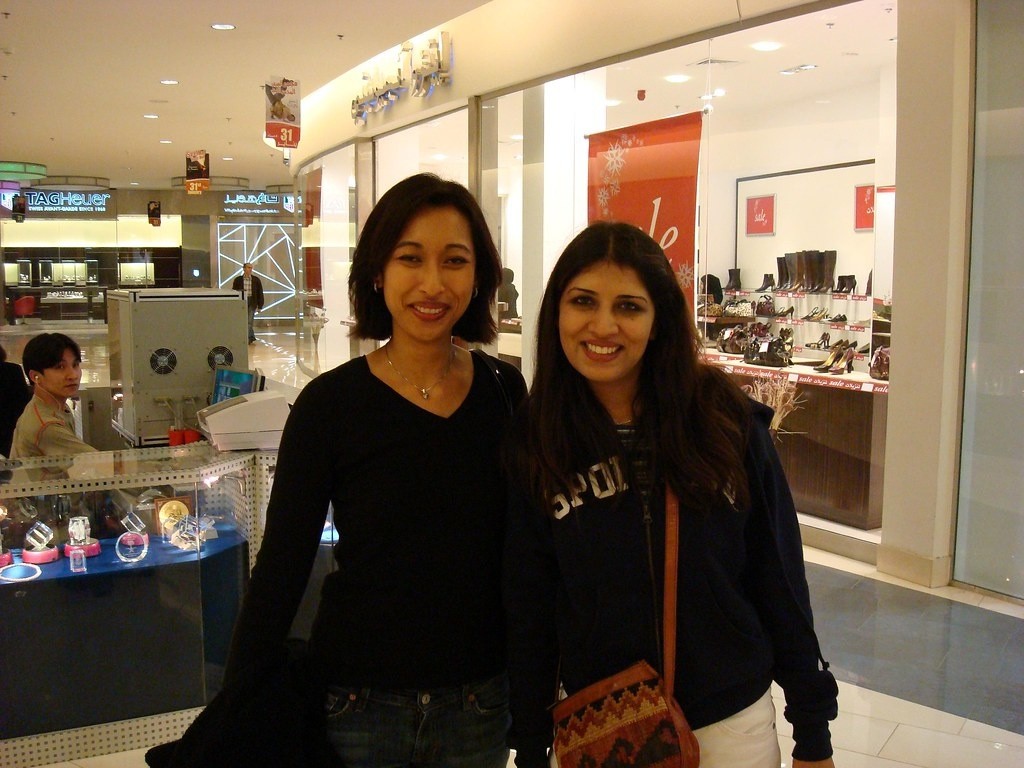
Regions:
[248,338,257,345]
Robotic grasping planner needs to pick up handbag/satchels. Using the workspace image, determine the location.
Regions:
[697,302,723,317]
[869,345,890,380]
[716,321,772,354]
[723,299,754,318]
[756,295,775,316]
[549,660,701,768]
[744,336,788,367]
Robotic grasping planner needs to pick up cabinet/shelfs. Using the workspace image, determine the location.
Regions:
[72,387,128,450]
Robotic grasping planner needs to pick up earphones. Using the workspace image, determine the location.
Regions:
[35,375,38,380]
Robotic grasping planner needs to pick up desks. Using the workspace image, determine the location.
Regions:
[5,285,108,326]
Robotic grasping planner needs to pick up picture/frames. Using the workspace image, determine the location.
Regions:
[745,193,776,238]
[854,182,876,232]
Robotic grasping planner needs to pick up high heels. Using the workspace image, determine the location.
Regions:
[778,305,846,322]
[780,328,794,349]
[813,345,854,375]
[828,340,870,353]
[805,332,830,349]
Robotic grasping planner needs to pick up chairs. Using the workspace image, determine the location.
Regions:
[498,267,522,334]
[13,295,36,325]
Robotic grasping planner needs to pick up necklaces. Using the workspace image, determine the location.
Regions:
[615,418,632,425]
[385,342,456,400]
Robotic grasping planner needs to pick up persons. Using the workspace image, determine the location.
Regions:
[498,268,518,318]
[223,173,528,768]
[232,263,264,345]
[700,274,723,305]
[265,78,295,123]
[0,333,99,484]
[504,222,838,768]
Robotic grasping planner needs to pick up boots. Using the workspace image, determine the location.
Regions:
[725,269,741,291]
[755,274,775,292]
[833,275,857,294]
[775,250,837,292]
